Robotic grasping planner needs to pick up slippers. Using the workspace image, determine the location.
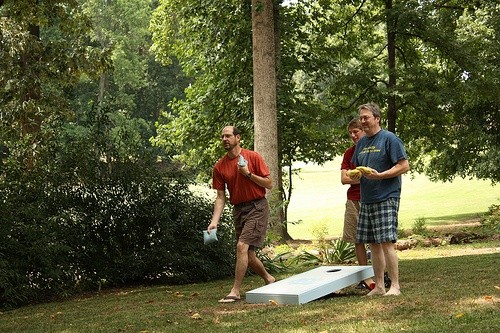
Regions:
[218,293,243,303]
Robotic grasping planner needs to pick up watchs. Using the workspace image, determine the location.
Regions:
[247,172,252,178]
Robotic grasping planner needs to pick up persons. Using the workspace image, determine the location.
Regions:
[206,125,277,304]
[340,116,391,289]
[346,103,410,298]
